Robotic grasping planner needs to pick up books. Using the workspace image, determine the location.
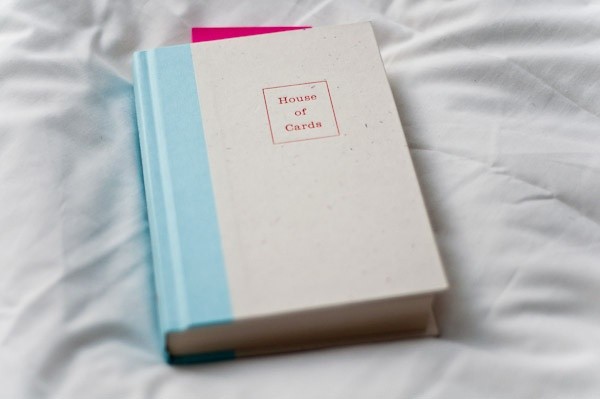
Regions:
[130,19,451,366]
